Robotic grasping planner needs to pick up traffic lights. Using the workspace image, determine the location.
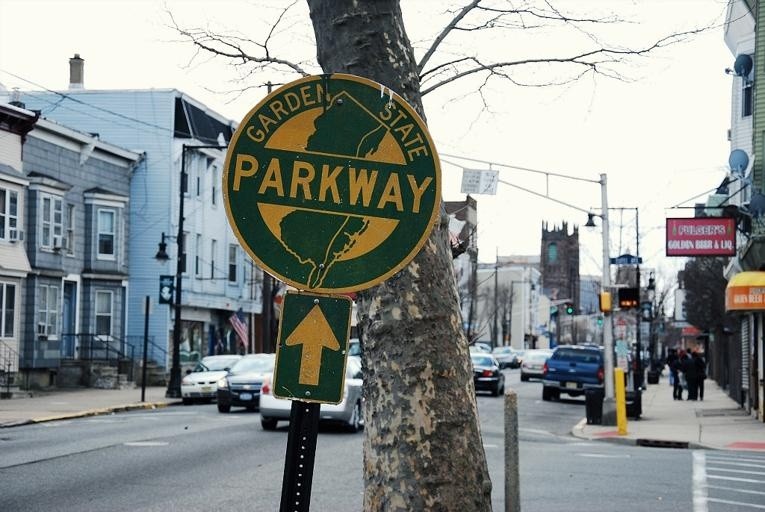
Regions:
[564,299,576,315]
[618,287,640,309]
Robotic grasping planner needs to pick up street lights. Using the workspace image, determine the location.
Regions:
[153,232,183,399]
[492,254,515,346]
[507,278,538,347]
[645,269,660,385]
[581,206,643,421]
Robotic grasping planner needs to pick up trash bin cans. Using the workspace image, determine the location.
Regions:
[648,371,658,383]
[585,385,605,424]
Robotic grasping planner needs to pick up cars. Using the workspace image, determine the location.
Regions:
[180,354,241,404]
[541,344,604,402]
[467,342,553,397]
[259,338,366,433]
[216,352,277,413]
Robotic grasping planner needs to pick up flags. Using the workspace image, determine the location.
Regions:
[228,309,250,348]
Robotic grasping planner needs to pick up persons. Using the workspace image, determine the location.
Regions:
[666,342,707,400]
